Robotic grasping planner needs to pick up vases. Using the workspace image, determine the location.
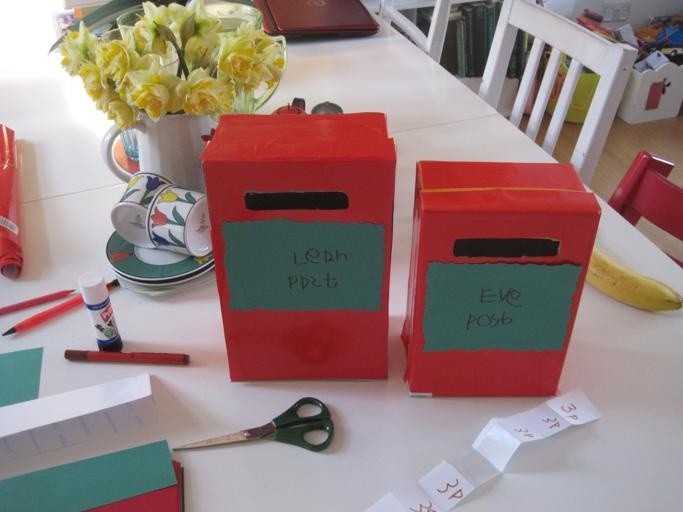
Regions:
[100,110,218,192]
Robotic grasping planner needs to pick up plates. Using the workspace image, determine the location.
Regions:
[106,231,214,287]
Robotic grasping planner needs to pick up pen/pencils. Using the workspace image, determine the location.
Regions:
[65,350,192,364]
[0,278,121,336]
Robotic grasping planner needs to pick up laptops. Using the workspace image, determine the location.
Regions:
[254,0,379,36]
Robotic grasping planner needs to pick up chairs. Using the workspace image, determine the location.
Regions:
[477,0,639,187]
[607,151,683,269]
[379,0,451,65]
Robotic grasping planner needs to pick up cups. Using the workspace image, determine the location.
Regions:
[110,172,176,249]
[146,184,212,257]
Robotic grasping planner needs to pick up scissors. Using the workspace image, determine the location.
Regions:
[172,397,336,452]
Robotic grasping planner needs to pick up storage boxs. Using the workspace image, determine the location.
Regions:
[543,54,601,124]
[615,62,683,125]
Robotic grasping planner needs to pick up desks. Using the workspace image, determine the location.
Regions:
[0,0,683,512]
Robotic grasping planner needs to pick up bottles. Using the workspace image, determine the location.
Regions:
[78,272,122,352]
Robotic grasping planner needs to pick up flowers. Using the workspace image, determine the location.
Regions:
[61,0,285,130]
